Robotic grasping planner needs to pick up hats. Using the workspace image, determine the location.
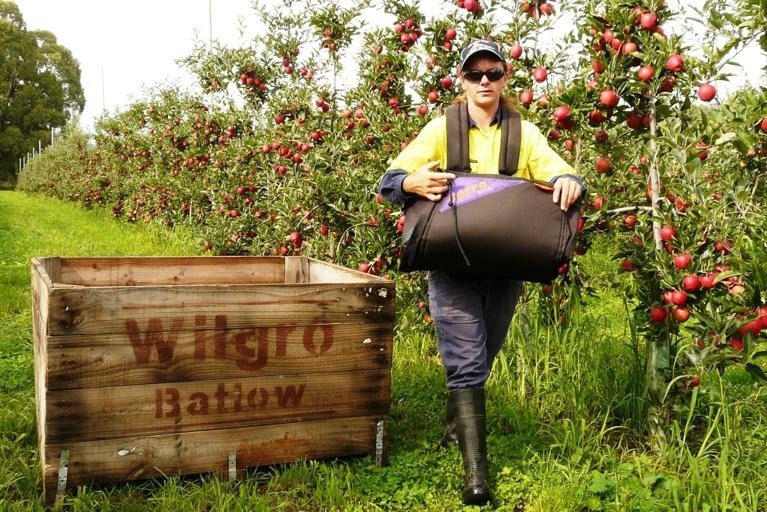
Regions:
[459,39,505,70]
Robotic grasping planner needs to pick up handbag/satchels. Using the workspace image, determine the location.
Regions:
[394,162,584,286]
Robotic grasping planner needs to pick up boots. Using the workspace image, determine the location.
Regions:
[444,385,493,506]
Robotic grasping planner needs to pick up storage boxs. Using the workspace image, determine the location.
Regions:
[31,252,397,509]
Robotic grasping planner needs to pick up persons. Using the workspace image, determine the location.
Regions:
[374,41,585,505]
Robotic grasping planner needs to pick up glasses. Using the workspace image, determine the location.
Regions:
[461,66,506,82]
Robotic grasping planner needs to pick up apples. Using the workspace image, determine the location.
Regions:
[75,0,767,388]
